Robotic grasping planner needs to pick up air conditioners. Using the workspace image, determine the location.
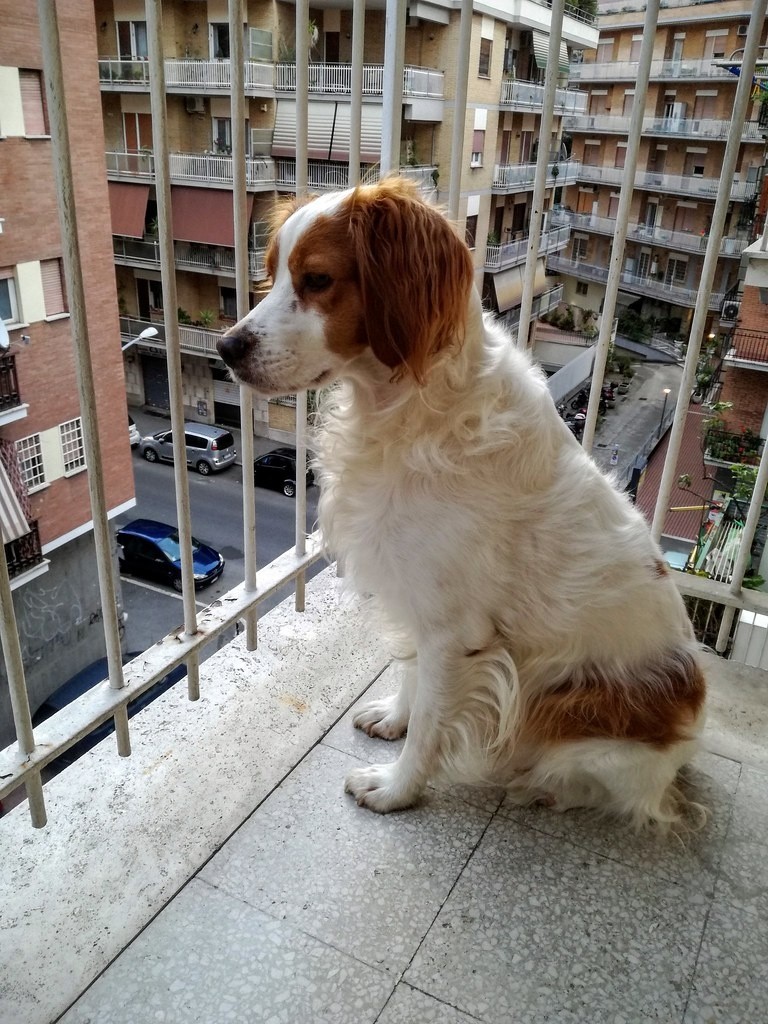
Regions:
[737,24,748,36]
[721,301,741,320]
[187,95,204,112]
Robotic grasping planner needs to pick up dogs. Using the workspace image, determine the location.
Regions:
[214,168,710,842]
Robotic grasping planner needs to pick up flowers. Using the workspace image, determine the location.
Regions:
[698,374,709,384]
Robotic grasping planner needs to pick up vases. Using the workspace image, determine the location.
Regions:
[691,393,703,403]
[701,386,705,394]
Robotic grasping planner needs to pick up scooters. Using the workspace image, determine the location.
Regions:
[561,367,636,442]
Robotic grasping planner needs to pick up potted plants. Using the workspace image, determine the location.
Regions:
[675,332,686,348]
[699,354,709,370]
[618,370,635,393]
[707,338,718,355]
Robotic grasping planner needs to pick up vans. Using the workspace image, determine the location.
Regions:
[115,517,224,593]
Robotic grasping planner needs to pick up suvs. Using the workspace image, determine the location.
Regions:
[140,421,238,476]
[252,447,315,497]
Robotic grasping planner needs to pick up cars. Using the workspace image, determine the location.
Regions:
[32,649,189,768]
[128,413,140,446]
